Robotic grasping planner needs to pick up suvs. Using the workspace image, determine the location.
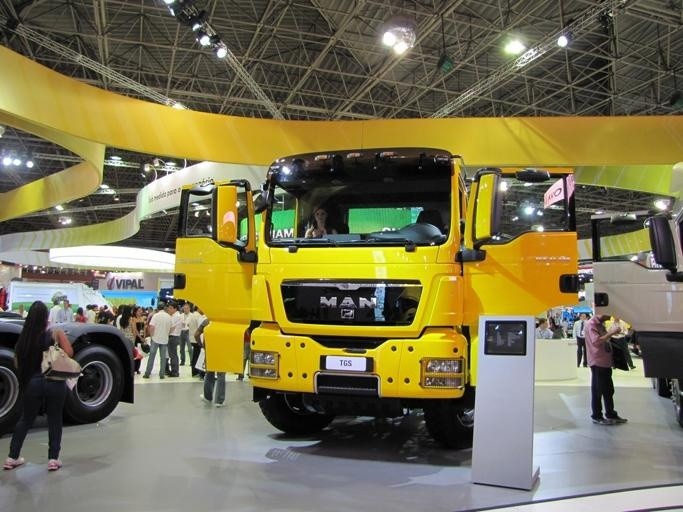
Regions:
[565,311,592,336]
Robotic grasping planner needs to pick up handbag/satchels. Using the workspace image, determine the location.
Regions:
[40,328,82,382]
[131,342,151,360]
[194,347,206,373]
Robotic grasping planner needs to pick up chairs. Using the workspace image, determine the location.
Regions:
[414,209,444,235]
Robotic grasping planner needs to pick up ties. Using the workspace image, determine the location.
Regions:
[581,321,584,331]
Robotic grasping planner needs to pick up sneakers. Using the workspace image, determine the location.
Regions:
[47,460,62,471]
[591,415,627,425]
[199,393,211,405]
[2,456,24,469]
[214,400,227,407]
[613,365,637,371]
[165,361,205,384]
[142,375,165,379]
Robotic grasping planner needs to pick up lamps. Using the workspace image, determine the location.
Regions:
[2,148,36,170]
[164,0,226,60]
[379,14,416,55]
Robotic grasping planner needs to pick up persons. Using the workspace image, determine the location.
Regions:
[2,302,74,471]
[585,313,628,425]
[50,297,257,406]
[534,304,640,370]
[305,203,337,238]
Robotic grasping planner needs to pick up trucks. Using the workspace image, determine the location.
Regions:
[7,280,114,319]
[164,140,589,451]
[589,157,681,431]
[150,287,184,308]
